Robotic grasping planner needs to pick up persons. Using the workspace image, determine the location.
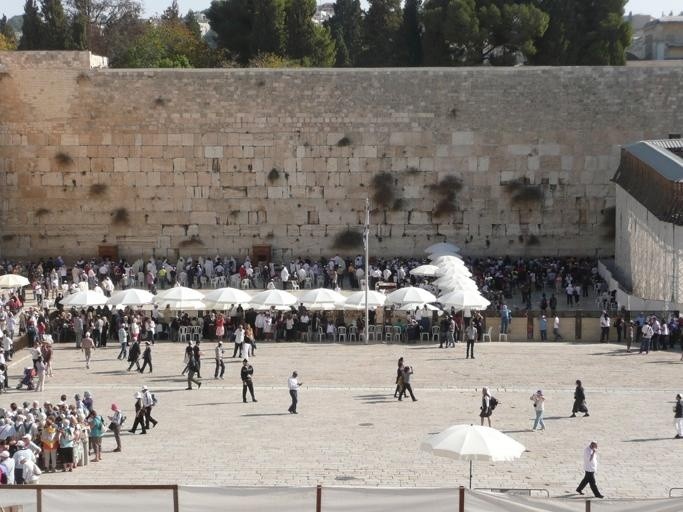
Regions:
[116,302,260,404]
[571,379,590,417]
[1,304,120,392]
[478,386,493,428]
[530,390,547,432]
[395,356,418,402]
[1,257,433,306]
[576,441,604,498]
[673,394,680,439]
[461,255,617,360]
[286,369,303,415]
[253,302,460,348]
[614,309,681,355]
[0,384,157,485]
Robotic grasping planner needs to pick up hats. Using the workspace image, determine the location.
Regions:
[0,385,149,465]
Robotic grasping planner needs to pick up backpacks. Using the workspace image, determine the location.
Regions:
[490,396,498,410]
